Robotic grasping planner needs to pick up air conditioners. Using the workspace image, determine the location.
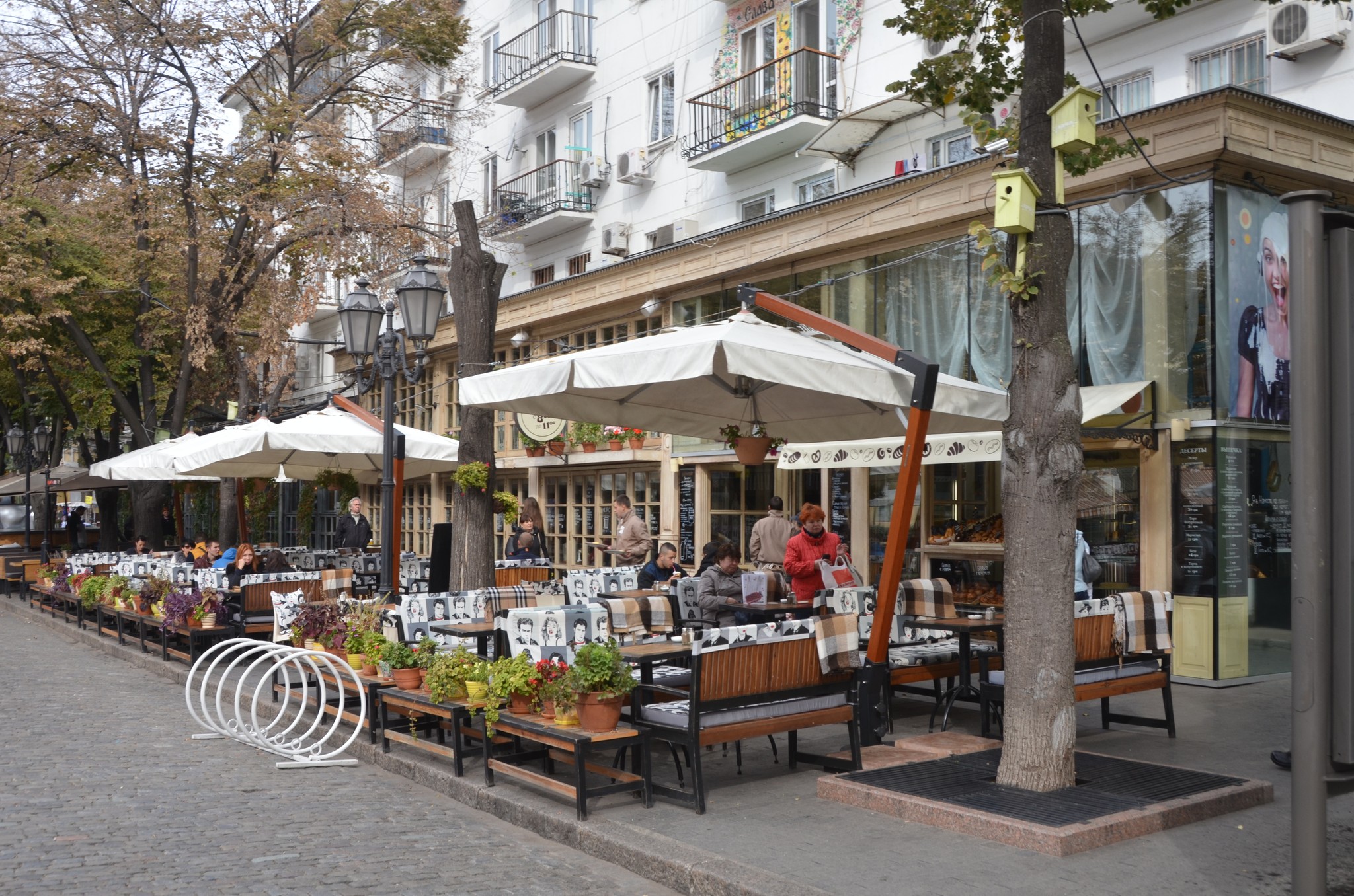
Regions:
[433,1,1354,253]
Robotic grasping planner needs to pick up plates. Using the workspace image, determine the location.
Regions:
[585,542,609,548]
[603,550,625,555]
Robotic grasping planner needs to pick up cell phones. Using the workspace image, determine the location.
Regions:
[822,554,831,560]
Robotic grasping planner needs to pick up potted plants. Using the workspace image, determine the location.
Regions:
[117,465,361,546]
[569,638,638,734]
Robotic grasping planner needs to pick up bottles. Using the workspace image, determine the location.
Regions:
[653,581,656,591]
[688,627,695,643]
[656,581,660,591]
[681,627,691,644]
[340,592,349,601]
[986,608,993,620]
[787,592,793,604]
[791,592,797,604]
[990,606,996,620]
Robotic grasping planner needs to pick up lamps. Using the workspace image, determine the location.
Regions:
[344,291,661,388]
[1144,189,1172,219]
[1110,175,1142,213]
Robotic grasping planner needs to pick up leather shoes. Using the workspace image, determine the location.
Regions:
[1271,750,1292,768]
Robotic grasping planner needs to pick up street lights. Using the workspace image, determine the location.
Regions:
[5,418,52,599]
[338,251,448,628]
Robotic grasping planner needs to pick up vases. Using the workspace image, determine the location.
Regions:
[511,690,533,709]
[493,497,509,514]
[393,668,424,690]
[465,679,488,698]
[733,438,773,465]
[524,437,645,457]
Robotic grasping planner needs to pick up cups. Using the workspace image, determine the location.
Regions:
[673,571,681,578]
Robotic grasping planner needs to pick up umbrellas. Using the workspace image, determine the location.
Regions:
[83,254,1173,751]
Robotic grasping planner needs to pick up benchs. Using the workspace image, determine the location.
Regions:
[0,545,353,667]
[271,546,1177,814]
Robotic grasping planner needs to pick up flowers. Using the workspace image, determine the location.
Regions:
[519,419,647,452]
[35,564,571,739]
[451,460,521,525]
[719,425,789,456]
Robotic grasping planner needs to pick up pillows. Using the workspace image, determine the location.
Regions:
[269,588,306,633]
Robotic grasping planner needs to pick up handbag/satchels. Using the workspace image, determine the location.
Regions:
[537,533,544,558]
[819,556,857,590]
[836,554,863,587]
[741,560,768,605]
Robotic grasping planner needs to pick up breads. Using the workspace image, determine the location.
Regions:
[926,516,1004,543]
[951,579,1004,605]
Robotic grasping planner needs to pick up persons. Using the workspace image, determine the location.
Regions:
[512,514,550,561]
[185,531,223,563]
[513,496,545,546]
[226,543,266,590]
[149,540,166,555]
[747,498,803,594]
[1234,211,1293,425]
[260,550,296,573]
[638,542,691,596]
[506,531,542,561]
[594,494,653,568]
[193,537,223,571]
[212,543,241,568]
[66,506,87,553]
[161,505,177,536]
[332,495,373,555]
[1070,525,1098,599]
[694,541,722,578]
[696,540,755,632]
[785,503,852,597]
[126,535,150,555]
[170,537,195,563]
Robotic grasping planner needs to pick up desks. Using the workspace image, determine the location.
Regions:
[429,622,497,657]
[598,589,673,597]
[900,616,1003,734]
[718,598,816,621]
[600,641,694,782]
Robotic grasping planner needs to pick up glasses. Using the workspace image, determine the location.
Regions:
[184,546,191,549]
